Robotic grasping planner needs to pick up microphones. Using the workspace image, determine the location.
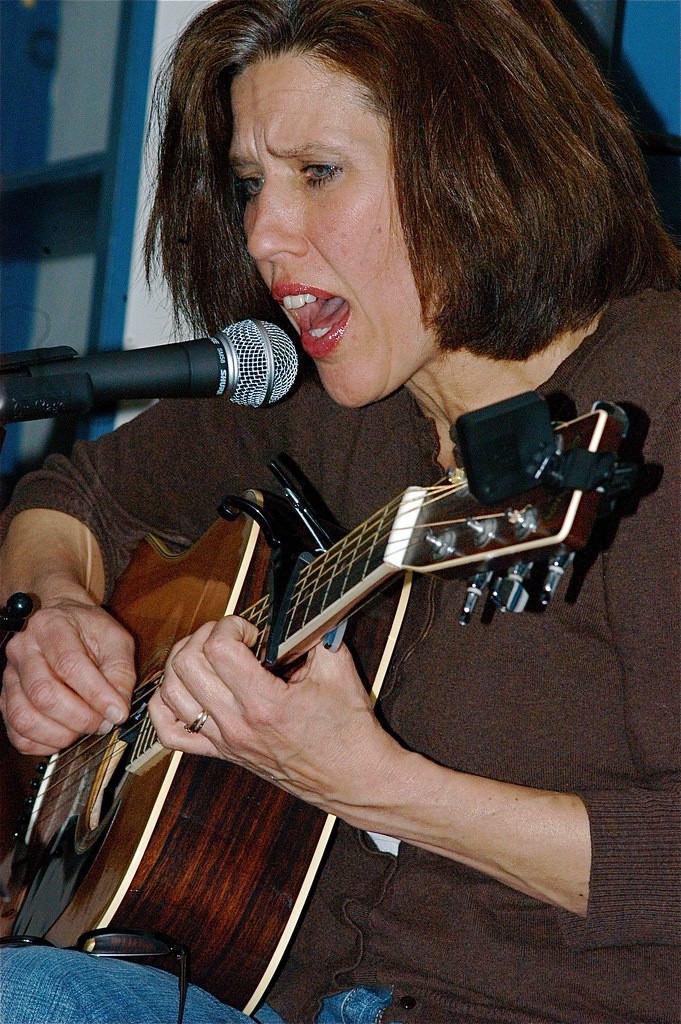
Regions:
[0,320,298,424]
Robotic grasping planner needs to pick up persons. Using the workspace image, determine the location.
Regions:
[0,0,681,1024]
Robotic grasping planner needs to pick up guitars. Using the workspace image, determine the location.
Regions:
[0,387,662,1015]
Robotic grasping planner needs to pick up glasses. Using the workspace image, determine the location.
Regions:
[0,926,188,1024]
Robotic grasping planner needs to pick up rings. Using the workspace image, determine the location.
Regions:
[186,711,209,734]
